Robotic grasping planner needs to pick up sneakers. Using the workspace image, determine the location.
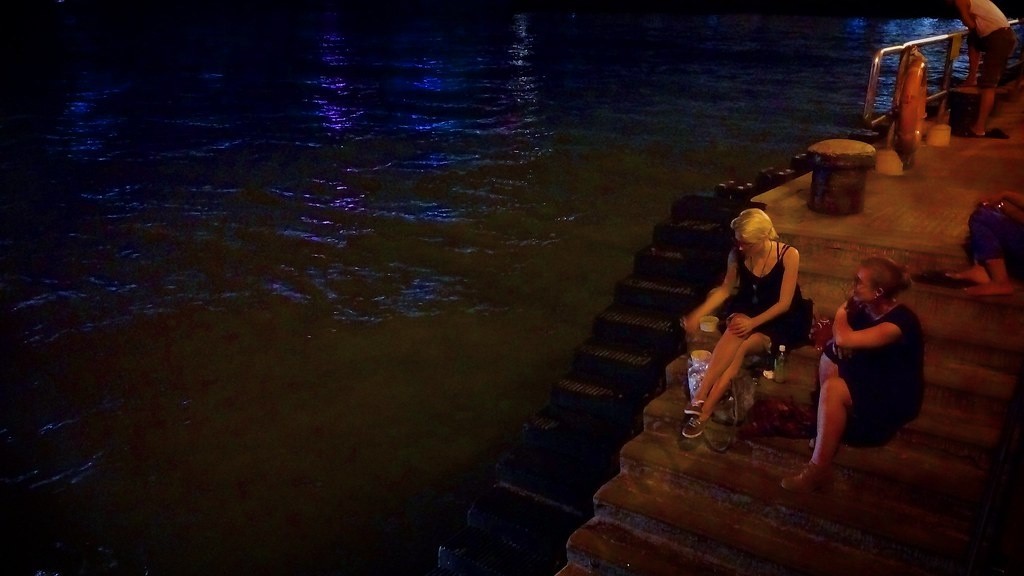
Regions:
[683,398,705,417]
[681,412,711,439]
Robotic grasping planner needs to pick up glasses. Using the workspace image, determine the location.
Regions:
[732,237,756,251]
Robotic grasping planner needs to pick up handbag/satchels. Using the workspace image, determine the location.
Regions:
[702,365,754,450]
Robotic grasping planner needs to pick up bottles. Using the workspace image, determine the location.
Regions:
[774,345,787,383]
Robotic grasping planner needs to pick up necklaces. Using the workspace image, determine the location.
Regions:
[749,240,772,291]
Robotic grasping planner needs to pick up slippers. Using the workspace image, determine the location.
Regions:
[985,124,1010,138]
[955,125,985,137]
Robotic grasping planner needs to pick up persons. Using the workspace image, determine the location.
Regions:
[678,208,807,437]
[943,189,1024,298]
[955,0,1017,138]
[781,255,925,494]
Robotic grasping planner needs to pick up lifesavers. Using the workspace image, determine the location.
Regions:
[897,56,927,153]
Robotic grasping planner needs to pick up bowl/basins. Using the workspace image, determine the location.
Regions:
[690,350,711,366]
[700,316,719,333]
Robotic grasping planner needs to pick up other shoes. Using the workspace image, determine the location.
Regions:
[778,461,835,495]
